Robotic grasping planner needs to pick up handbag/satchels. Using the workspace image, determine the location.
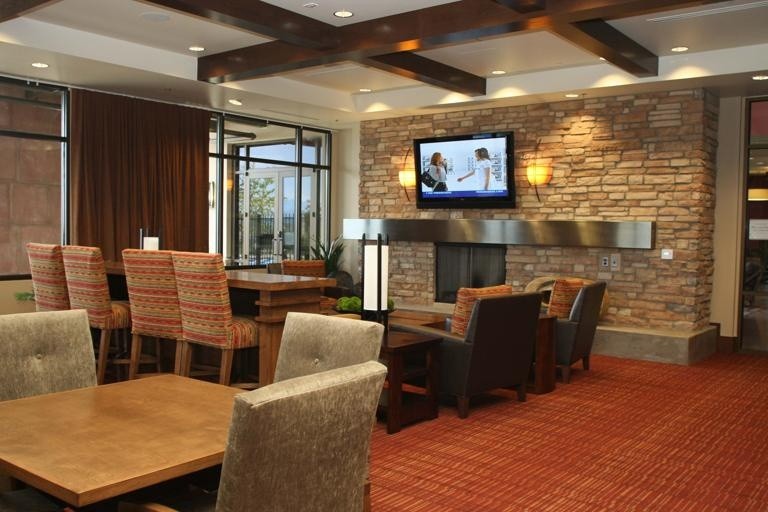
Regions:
[421,168,437,187]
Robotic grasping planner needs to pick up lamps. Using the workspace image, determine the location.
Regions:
[525,138,553,203]
[397,146,416,203]
[360,232,388,329]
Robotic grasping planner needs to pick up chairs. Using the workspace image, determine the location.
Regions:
[61,245,161,385]
[281,259,338,309]
[742,260,765,306]
[122,248,220,381]
[115,359,388,511]
[2,490,75,510]
[0,311,101,489]
[170,250,259,389]
[271,311,387,512]
[24,241,122,381]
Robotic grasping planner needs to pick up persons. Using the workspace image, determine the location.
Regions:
[456,147,492,191]
[442,159,448,175]
[428,152,448,192]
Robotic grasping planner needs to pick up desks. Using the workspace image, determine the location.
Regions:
[0,373,251,508]
[100,255,339,388]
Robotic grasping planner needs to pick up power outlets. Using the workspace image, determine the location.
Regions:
[601,256,609,268]
[610,253,618,269]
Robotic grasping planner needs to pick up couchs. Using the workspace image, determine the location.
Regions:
[540,281,606,384]
[388,292,545,419]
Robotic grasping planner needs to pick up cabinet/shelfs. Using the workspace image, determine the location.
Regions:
[374,331,444,435]
[515,314,559,396]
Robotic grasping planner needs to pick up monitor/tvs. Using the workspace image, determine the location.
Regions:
[412,131,516,209]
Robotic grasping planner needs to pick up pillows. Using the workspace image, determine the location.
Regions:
[451,284,513,340]
[546,278,584,319]
